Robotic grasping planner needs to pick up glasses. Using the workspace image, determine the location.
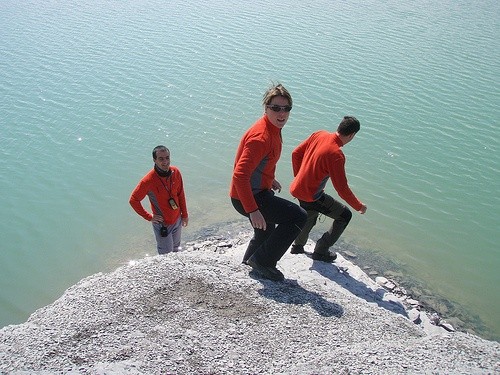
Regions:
[265,102,292,112]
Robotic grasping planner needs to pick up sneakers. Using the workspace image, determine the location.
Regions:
[249,255,285,282]
[316,247,338,263]
[290,245,305,254]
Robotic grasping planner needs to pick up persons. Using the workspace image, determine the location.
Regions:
[230,84,309,282]
[289,116,367,262]
[129,145,188,255]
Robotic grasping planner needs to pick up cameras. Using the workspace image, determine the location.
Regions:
[168,198,178,209]
